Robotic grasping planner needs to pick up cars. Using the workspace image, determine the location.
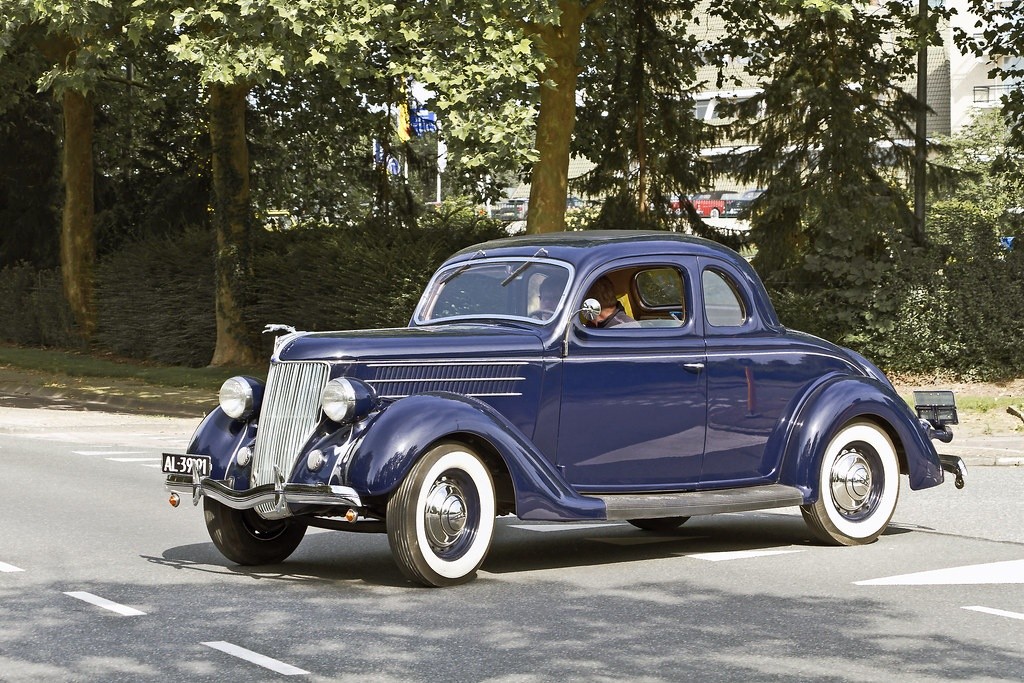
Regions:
[499,197,530,221]
[157,230,969,588]
[648,189,765,218]
[566,196,585,209]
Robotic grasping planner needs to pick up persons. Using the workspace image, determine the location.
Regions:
[535,275,567,320]
[581,275,641,328]
